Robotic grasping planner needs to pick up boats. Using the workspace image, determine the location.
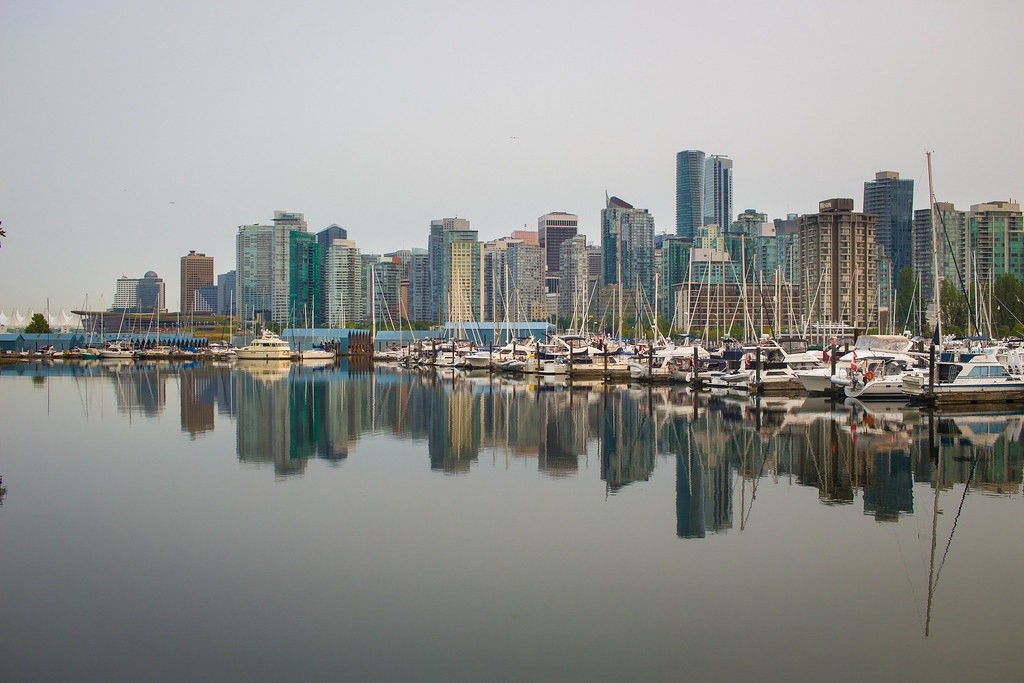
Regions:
[234,328,290,360]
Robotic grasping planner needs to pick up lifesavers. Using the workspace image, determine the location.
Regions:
[686,373,695,383]
[865,371,874,380]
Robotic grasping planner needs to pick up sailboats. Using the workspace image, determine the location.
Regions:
[373,152,1024,399]
[297,295,336,359]
[2,284,237,358]
